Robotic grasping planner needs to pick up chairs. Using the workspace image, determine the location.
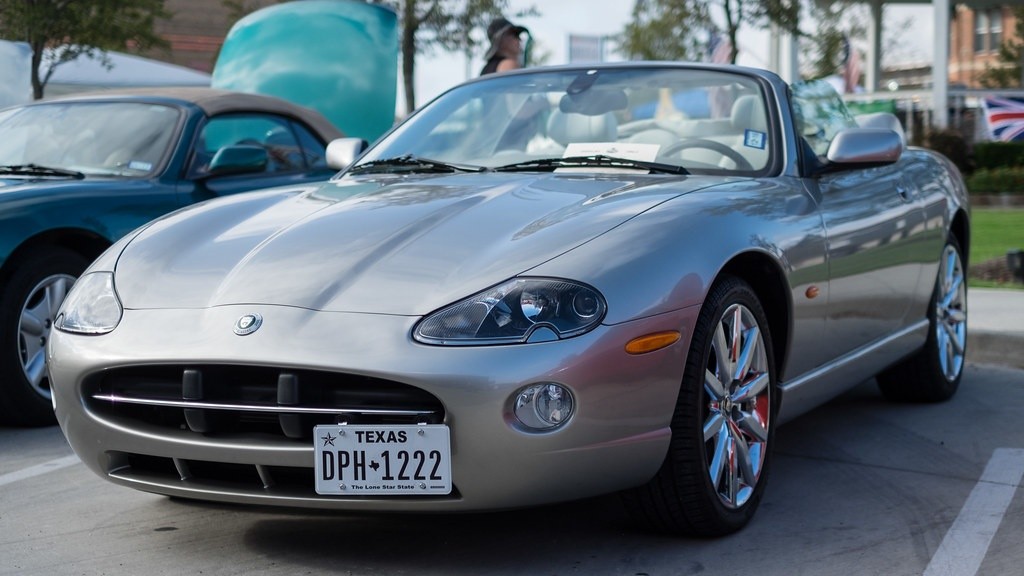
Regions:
[718,95,770,170]
[546,107,618,156]
[625,129,682,159]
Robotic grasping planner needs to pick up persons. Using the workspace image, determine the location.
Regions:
[476,15,547,156]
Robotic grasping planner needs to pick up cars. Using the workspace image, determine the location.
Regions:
[171,1,470,179]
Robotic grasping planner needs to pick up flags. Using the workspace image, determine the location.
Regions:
[983,91,1024,144]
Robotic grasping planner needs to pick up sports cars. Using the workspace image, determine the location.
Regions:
[45,59,973,541]
[0,86,370,431]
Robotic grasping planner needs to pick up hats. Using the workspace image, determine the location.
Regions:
[484,17,530,60]
